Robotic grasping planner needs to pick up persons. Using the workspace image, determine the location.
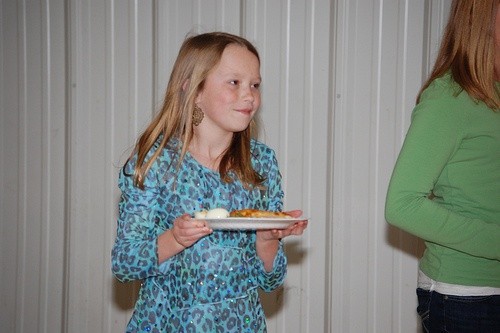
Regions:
[111,32,309,333]
[385,0,500,333]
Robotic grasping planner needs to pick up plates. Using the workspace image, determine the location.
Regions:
[192,216,307,230]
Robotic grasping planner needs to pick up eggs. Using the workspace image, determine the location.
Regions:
[204,208,231,219]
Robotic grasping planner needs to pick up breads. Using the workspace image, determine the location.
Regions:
[229,209,291,218]
